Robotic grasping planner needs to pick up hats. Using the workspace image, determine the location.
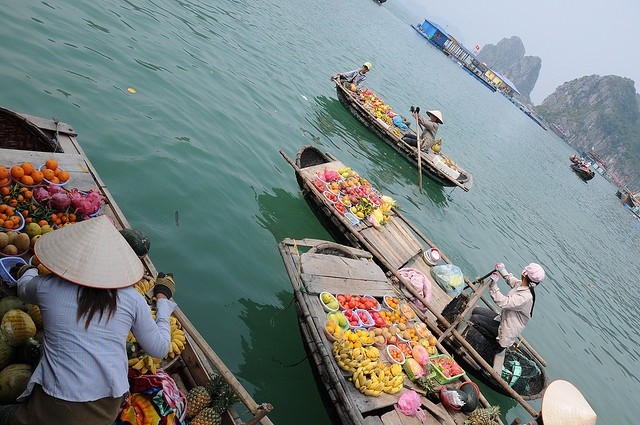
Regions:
[363,61,373,71]
[541,379,597,425]
[426,110,443,123]
[33,214,145,288]
[522,262,546,282]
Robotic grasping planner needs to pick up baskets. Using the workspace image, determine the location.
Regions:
[403,356,417,382]
[0,256,29,284]
[428,353,465,385]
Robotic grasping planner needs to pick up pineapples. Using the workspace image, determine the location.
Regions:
[382,195,395,205]
[189,386,239,425]
[1,309,41,356]
[373,211,385,225]
[185,376,228,420]
[413,345,431,369]
[26,302,42,329]
[463,404,502,425]
[409,358,426,378]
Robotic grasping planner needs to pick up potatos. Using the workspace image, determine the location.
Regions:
[369,323,409,343]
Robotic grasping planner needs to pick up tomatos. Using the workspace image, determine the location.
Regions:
[335,203,345,213]
[356,186,378,205]
[313,180,323,192]
[325,191,336,203]
[338,294,383,327]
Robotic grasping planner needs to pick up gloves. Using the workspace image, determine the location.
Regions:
[415,106,420,113]
[9,262,40,280]
[489,271,499,290]
[153,271,176,299]
[410,105,415,112]
[495,261,509,276]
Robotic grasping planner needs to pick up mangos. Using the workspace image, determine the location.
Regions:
[357,212,364,218]
[327,323,369,348]
[352,206,357,214]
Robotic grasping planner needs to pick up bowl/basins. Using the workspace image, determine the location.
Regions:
[2,256,29,287]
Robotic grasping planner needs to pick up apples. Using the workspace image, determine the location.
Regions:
[321,293,338,310]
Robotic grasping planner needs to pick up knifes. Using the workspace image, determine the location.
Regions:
[31,188,45,208]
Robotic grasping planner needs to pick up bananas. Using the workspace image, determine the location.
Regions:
[127,356,162,375]
[333,342,403,396]
[134,280,157,303]
[164,316,186,360]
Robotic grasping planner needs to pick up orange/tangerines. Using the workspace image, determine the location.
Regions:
[0,151,70,187]
[50,212,77,228]
[0,187,48,228]
[407,328,419,348]
[380,310,406,323]
[386,295,399,310]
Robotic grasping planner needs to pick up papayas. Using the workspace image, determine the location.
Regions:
[328,312,348,329]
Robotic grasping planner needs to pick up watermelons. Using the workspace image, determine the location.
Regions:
[462,384,478,412]
[119,229,150,256]
[444,387,468,409]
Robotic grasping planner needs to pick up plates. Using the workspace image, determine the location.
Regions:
[382,294,399,312]
[323,319,344,343]
[158,355,181,369]
[1,210,26,232]
[338,197,354,208]
[441,386,462,412]
[316,174,330,186]
[145,276,168,300]
[320,291,340,313]
[0,164,13,188]
[367,194,380,207]
[348,206,365,219]
[78,190,102,217]
[9,164,41,189]
[353,326,375,347]
[323,190,338,205]
[368,326,387,348]
[1,231,31,257]
[350,293,362,309]
[392,323,410,343]
[38,163,71,186]
[387,343,406,368]
[423,248,442,267]
[334,202,347,216]
[327,310,350,333]
[370,186,383,198]
[326,182,340,197]
[334,293,354,312]
[458,381,480,401]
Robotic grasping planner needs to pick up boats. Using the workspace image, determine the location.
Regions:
[570,163,595,181]
[278,238,509,425]
[0,106,275,425]
[279,145,549,400]
[616,189,634,208]
[331,75,473,192]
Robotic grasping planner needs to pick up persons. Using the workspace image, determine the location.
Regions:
[17,213,177,425]
[400,105,444,156]
[333,56,372,97]
[471,261,548,355]
